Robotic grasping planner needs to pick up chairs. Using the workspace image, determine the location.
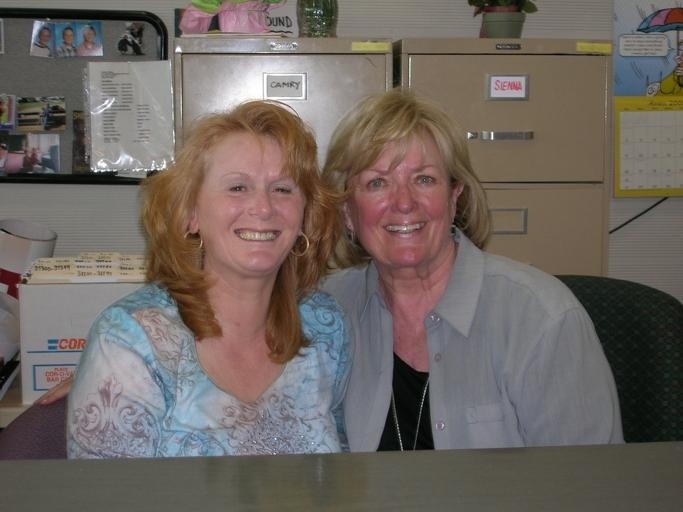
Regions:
[0,387,70,459]
[548,274,682,444]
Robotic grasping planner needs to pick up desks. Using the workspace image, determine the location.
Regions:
[0,440,683,509]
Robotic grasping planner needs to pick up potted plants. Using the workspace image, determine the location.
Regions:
[466,1,538,39]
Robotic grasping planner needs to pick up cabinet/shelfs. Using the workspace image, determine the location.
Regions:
[458,184,614,279]
[167,34,397,186]
[392,33,615,184]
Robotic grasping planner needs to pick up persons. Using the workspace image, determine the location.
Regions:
[77,24,102,56]
[65,99,352,461]
[41,108,52,131]
[46,103,51,112]
[23,148,39,171]
[31,25,52,57]
[34,87,625,452]
[118,35,132,56]
[57,26,77,57]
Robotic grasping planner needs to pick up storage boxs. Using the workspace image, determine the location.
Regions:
[13,270,164,405]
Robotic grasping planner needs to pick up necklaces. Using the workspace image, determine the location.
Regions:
[392,376,430,451]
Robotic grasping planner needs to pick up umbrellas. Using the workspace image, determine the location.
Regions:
[636,7,683,87]
